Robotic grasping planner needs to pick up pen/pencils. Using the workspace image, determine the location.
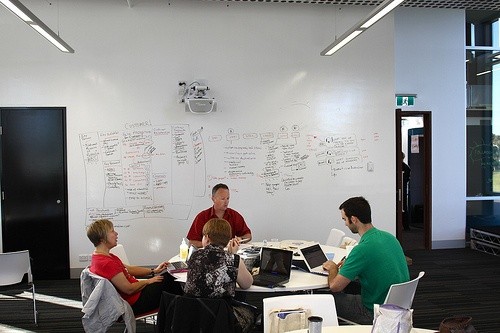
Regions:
[341,256,346,261]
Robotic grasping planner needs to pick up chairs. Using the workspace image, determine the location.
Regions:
[384,271,425,308]
[86,241,238,333]
[0,249,37,326]
[263,294,339,333]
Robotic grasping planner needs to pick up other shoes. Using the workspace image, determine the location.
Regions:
[403,225,410,232]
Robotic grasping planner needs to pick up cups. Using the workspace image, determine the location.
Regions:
[346,246,353,256]
[308,316,323,333]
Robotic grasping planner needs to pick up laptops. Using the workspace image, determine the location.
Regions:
[252,247,294,288]
[167,244,200,272]
[299,243,339,276]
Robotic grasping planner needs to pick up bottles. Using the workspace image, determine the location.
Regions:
[180,238,189,261]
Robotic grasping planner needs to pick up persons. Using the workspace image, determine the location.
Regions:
[187,184,252,248]
[322,197,410,325]
[402,153,411,230]
[184,218,257,333]
[87,219,183,316]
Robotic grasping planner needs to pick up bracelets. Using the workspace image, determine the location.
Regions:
[151,268,154,276]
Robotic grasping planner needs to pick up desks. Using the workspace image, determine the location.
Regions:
[167,241,347,295]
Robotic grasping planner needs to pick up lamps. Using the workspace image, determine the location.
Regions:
[320,0,405,56]
[0,0,74,53]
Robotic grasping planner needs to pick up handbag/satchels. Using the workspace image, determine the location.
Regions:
[371,303,414,333]
[267,307,311,333]
